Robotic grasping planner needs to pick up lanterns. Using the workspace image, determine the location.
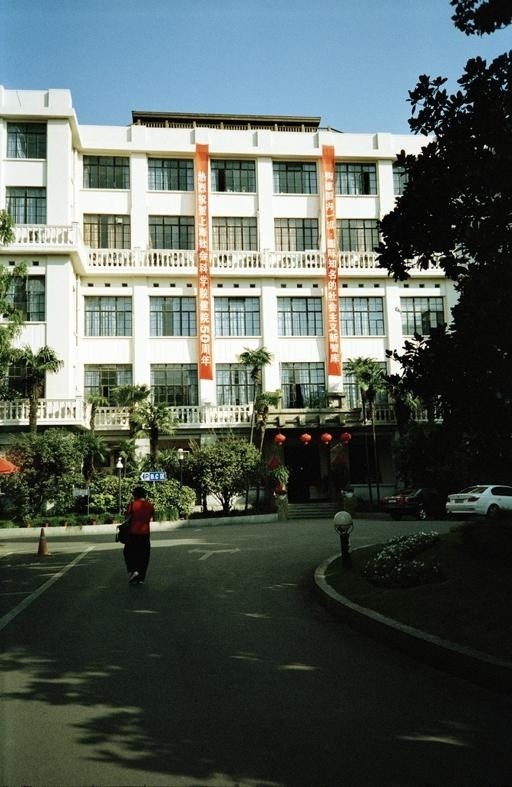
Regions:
[274,433,286,446]
[300,433,311,445]
[320,432,332,445]
[340,432,352,445]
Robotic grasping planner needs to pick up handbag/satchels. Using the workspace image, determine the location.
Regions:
[115,518,130,543]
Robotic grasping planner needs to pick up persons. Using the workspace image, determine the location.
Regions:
[122,487,154,585]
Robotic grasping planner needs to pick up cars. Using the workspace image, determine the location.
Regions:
[445,484,512,517]
[382,484,447,521]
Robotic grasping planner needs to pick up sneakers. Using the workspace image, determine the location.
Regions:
[128,569,144,585]
